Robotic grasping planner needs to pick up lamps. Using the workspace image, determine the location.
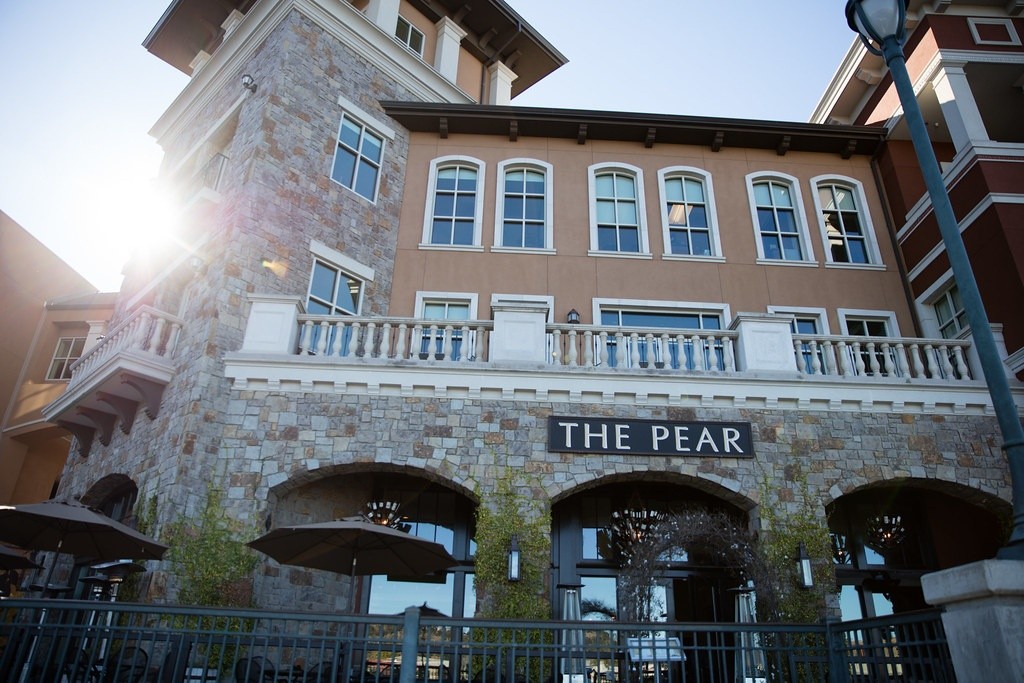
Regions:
[612,508,677,571]
[863,516,904,551]
[506,540,523,583]
[95,333,105,345]
[565,306,581,326]
[797,541,815,588]
[668,204,693,225]
[358,500,410,533]
[241,73,255,93]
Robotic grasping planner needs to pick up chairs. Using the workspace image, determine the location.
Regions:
[67,645,172,683]
[236,656,342,683]
[475,670,505,683]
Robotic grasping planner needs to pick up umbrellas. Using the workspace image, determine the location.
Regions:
[242,521,459,683]
[0,547,49,597]
[395,599,453,665]
[0,490,172,683]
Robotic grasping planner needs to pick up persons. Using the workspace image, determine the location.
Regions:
[586,667,597,683]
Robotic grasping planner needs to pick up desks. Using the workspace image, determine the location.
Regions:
[257,669,324,683]
[95,659,158,683]
